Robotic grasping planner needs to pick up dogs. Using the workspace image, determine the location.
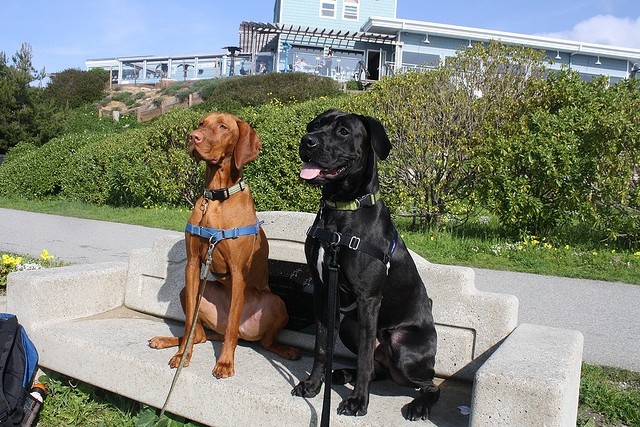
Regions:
[147,111,301,380]
[290,108,440,422]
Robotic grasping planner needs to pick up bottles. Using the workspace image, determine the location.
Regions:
[20,383,46,427]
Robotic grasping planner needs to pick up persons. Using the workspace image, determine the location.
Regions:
[295,58,311,71]
[254,62,266,75]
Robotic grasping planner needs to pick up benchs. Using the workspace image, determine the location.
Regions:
[6,211,584,427]
